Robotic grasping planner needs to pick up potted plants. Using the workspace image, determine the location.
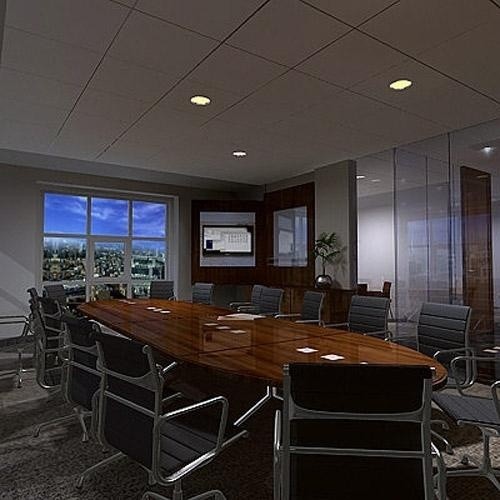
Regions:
[306,231,348,288]
